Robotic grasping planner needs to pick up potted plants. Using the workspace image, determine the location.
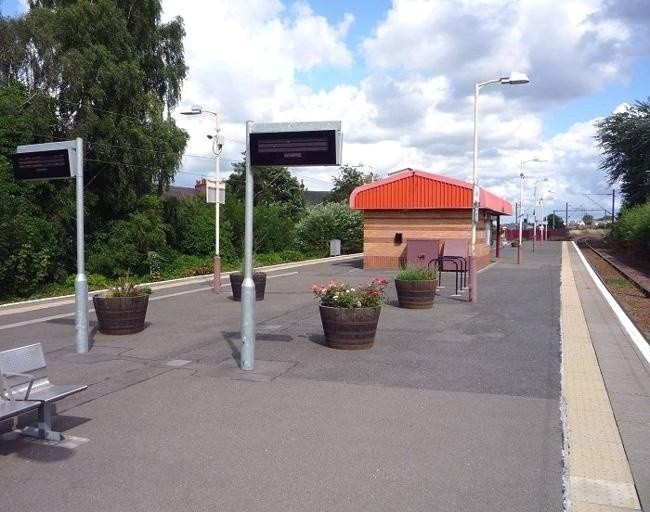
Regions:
[229,258,266,301]
[93,268,152,336]
[394,264,438,309]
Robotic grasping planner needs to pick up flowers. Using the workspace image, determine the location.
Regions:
[310,278,390,308]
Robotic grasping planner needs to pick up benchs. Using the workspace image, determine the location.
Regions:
[0,343,87,441]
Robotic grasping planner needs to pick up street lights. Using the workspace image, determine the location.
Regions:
[515,155,555,268]
[179,101,226,292]
[468,72,533,306]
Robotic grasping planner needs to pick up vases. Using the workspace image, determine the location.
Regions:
[318,302,381,350]
